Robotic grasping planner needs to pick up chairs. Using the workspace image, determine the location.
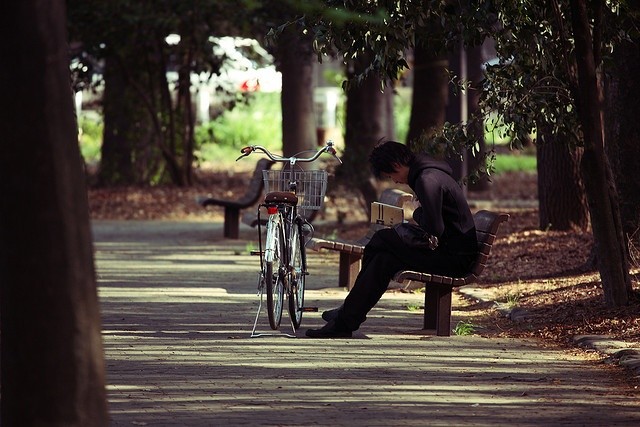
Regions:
[197,157,276,239]
[387,210,511,336]
[300,187,414,293]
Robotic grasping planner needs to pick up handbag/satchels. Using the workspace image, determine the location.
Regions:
[391,223,439,251]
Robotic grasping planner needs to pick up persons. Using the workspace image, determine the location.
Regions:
[305,141,478,336]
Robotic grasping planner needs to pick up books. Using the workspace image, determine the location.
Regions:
[367,199,409,228]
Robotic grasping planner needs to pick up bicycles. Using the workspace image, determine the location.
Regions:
[236,139,342,338]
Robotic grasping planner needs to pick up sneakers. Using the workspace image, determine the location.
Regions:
[322,304,366,331]
[305,318,352,338]
